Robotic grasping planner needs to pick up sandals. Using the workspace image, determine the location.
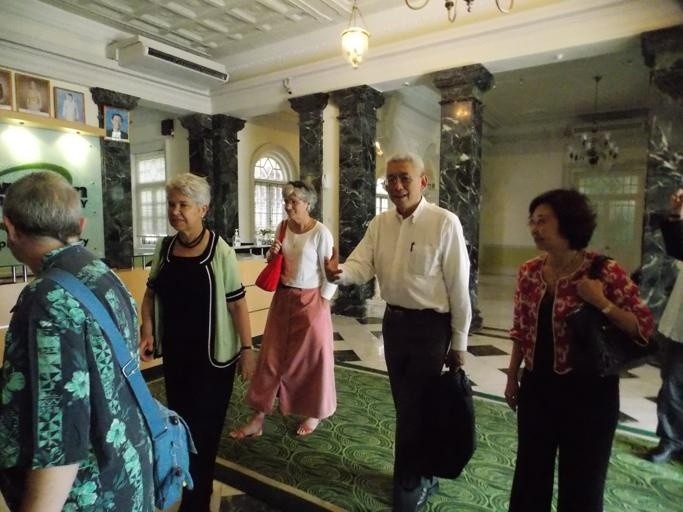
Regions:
[296,416,322,436]
[228,417,263,440]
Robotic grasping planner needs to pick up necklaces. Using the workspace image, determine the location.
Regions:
[174,228,206,249]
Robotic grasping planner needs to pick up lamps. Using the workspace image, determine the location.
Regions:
[405,0,514,23]
[564,75,619,165]
[339,0,370,68]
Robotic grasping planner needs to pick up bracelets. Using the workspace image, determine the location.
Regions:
[600,302,616,313]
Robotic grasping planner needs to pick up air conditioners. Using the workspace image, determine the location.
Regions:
[106,34,229,90]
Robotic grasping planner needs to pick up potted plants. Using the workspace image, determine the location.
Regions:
[267,229,275,239]
[260,229,267,238]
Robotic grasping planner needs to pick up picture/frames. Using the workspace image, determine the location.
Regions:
[13,70,52,117]
[0,67,13,110]
[53,86,85,125]
[103,106,130,143]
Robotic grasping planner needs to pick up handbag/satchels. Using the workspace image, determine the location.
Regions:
[563,254,660,379]
[255,220,288,291]
[140,237,172,359]
[421,369,475,480]
[39,268,198,512]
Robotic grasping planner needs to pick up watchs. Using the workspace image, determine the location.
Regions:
[238,345,257,349]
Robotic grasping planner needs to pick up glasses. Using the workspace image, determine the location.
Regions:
[287,181,309,192]
[383,175,413,187]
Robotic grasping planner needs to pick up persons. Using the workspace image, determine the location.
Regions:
[61,92,80,121]
[138,178,255,507]
[0,172,154,510]
[228,182,333,441]
[324,155,472,512]
[644,186,683,463]
[504,188,653,512]
[106,113,127,140]
[24,80,41,113]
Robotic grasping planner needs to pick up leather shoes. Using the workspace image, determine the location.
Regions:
[645,438,679,463]
[417,476,439,509]
[391,485,420,512]
[670,448,682,464]
